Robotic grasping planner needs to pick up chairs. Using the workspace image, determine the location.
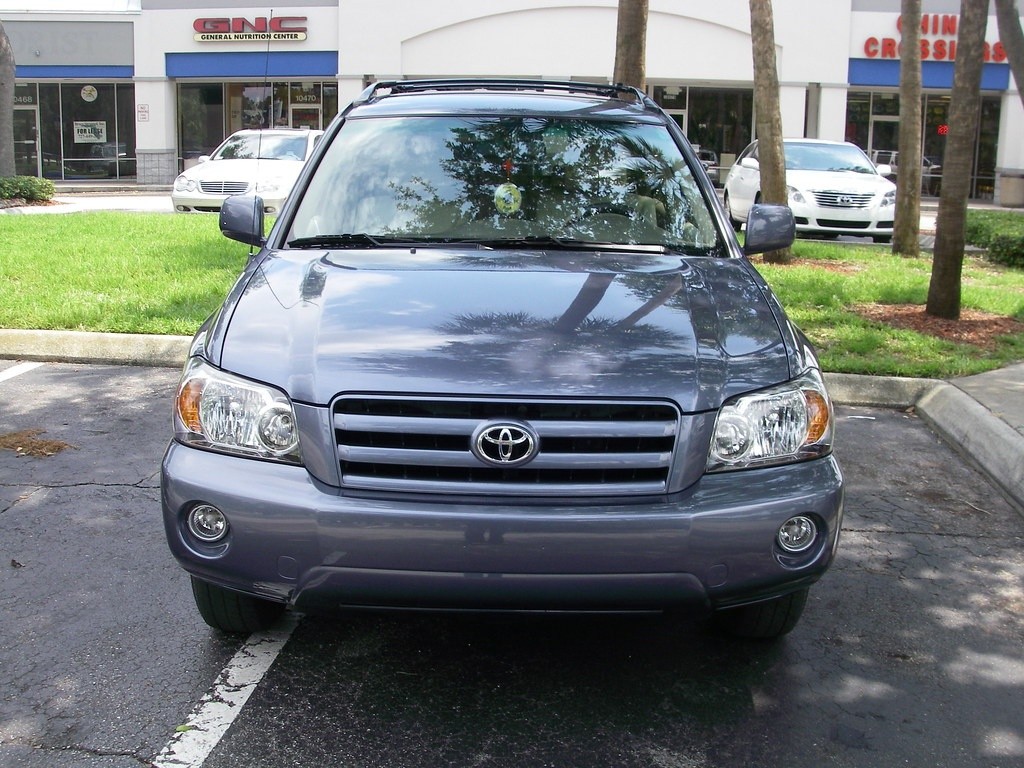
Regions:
[535,138,657,227]
[352,150,470,234]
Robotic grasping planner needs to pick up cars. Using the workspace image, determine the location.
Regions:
[865,148,943,197]
[694,149,719,189]
[170,128,325,214]
[157,77,847,635]
[722,136,897,244]
[87,143,126,172]
